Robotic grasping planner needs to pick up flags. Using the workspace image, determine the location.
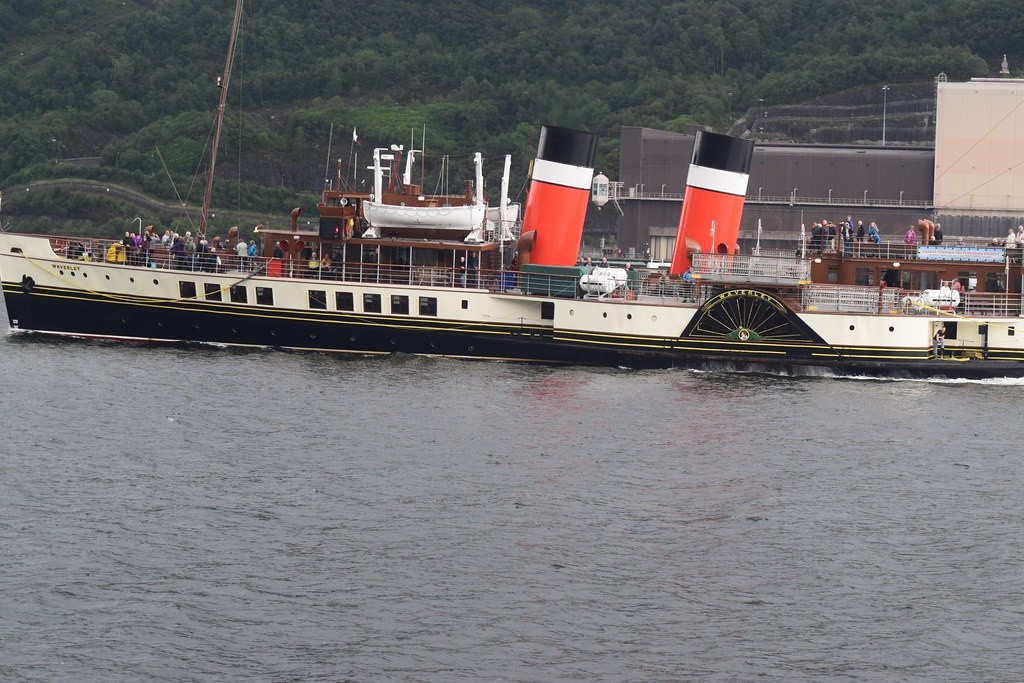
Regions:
[352,130,360,145]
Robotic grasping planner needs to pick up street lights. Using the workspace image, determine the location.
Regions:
[758,98,764,142]
[882,85,891,146]
[728,93,733,137]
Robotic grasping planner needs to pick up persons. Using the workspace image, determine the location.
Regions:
[124,231,160,267]
[657,270,670,295]
[236,239,248,270]
[576,256,596,266]
[249,240,256,270]
[934,223,942,241]
[955,237,965,247]
[1004,225,1024,248]
[467,252,479,288]
[598,257,609,267]
[682,268,695,303]
[932,327,946,358]
[623,262,633,271]
[809,215,880,258]
[162,230,217,272]
[903,225,916,244]
[224,239,229,247]
[929,236,938,245]
[673,272,684,296]
[942,278,961,290]
[308,253,331,275]
[458,256,466,287]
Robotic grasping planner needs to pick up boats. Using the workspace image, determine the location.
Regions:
[361,198,486,242]
[484,202,519,238]
[0,0,1024,391]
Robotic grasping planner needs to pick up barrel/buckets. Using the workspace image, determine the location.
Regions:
[268,259,281,277]
